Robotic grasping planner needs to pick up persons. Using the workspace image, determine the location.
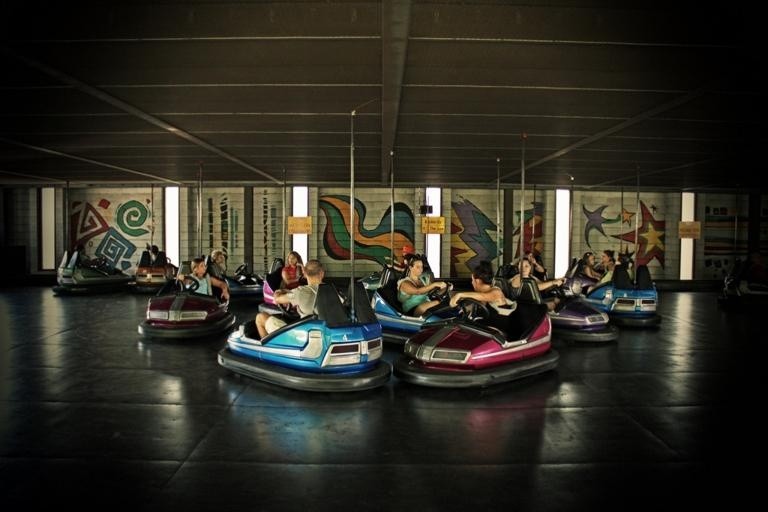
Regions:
[177,257,230,300]
[581,249,602,281]
[255,259,325,339]
[396,257,454,317]
[524,250,545,273]
[209,249,224,279]
[448,260,518,317]
[279,251,305,289]
[594,250,614,273]
[587,251,635,295]
[508,257,567,311]
